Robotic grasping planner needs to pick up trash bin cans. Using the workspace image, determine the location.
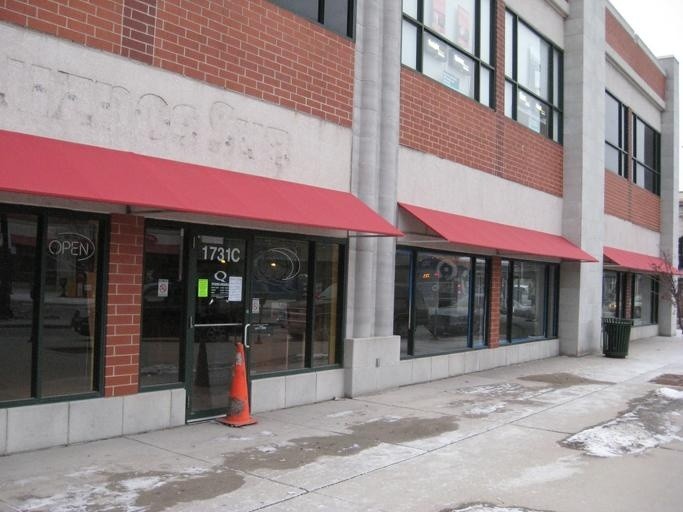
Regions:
[601,316,633,358]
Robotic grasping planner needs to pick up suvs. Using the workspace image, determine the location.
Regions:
[285,280,429,344]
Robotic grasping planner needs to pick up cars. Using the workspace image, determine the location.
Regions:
[610,296,642,319]
[425,293,535,336]
[73,276,260,340]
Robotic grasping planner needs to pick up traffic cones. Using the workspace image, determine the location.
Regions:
[189,340,213,414]
[216,339,261,428]
